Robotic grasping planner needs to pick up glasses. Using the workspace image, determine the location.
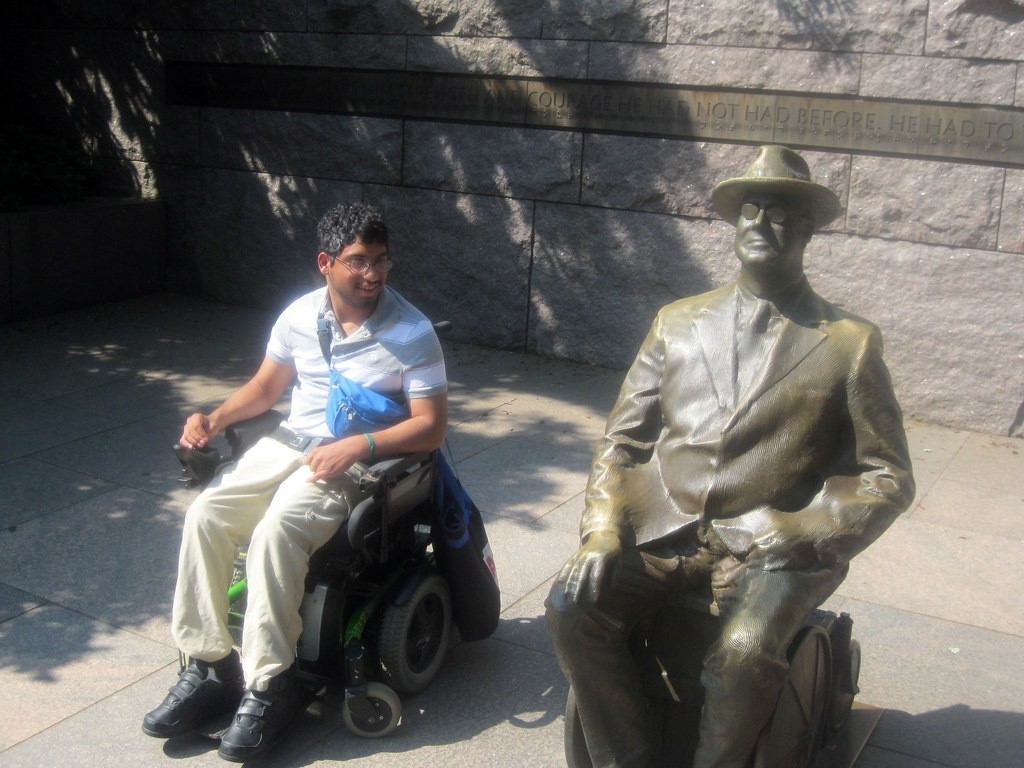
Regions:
[328,253,393,274]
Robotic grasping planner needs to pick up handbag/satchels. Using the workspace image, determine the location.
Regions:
[436,450,501,642]
[328,367,410,440]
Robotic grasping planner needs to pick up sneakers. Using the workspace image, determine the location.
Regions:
[142,649,242,738]
[218,664,300,762]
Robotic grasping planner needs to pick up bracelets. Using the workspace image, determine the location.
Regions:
[364,433,377,460]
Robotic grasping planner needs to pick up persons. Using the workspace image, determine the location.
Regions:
[545,146,917,768]
[141,202,448,763]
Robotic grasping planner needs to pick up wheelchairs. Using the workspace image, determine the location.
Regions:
[173,319,453,738]
